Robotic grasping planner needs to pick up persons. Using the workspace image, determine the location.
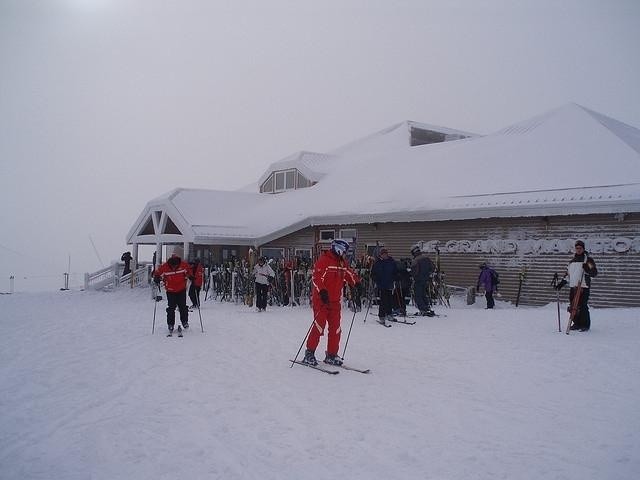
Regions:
[476,263,499,309]
[304,240,362,366]
[409,246,435,315]
[371,249,398,323]
[151,251,156,276]
[122,252,132,276]
[253,256,275,312]
[392,261,411,316]
[189,257,205,309]
[153,255,195,331]
[555,241,598,332]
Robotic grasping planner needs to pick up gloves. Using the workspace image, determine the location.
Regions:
[354,282,362,296]
[189,276,196,281]
[319,289,328,304]
[154,276,160,283]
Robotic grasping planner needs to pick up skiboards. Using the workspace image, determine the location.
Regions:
[166,326,183,338]
[291,358,370,374]
[378,317,415,327]
[566,252,592,335]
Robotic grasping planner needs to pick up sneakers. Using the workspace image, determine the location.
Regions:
[168,324,174,331]
[379,308,407,324]
[304,350,318,366]
[325,352,344,366]
[183,323,189,328]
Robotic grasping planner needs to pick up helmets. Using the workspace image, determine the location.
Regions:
[330,239,350,259]
[410,244,420,254]
